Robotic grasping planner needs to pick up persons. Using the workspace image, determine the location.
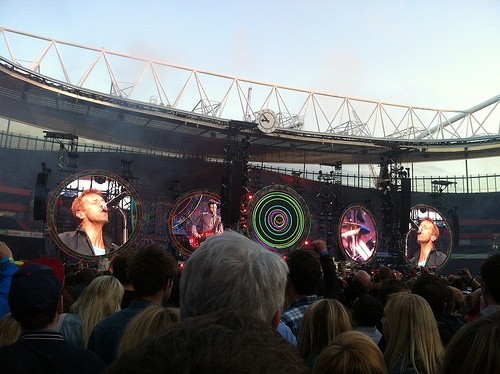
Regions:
[0,226,499,374]
[407,216,447,267]
[57,188,122,257]
[192,199,223,239]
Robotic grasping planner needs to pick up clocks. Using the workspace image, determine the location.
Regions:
[256,109,279,134]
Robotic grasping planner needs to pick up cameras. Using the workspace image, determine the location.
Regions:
[457,269,467,275]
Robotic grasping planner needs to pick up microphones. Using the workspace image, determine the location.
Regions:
[103,191,126,211]
[409,219,421,234]
[211,201,220,204]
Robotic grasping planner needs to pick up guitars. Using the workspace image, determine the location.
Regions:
[189,231,225,250]
